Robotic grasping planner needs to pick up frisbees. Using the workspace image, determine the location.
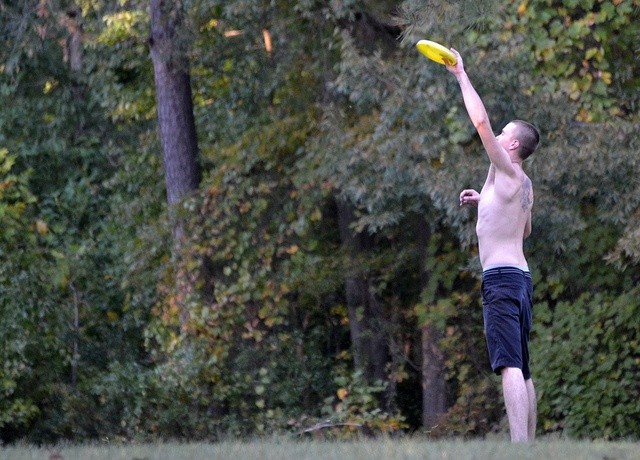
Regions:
[416,39,458,67]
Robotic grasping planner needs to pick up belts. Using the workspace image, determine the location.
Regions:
[482,269,530,278]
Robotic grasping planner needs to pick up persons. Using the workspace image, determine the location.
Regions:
[442,47,541,442]
